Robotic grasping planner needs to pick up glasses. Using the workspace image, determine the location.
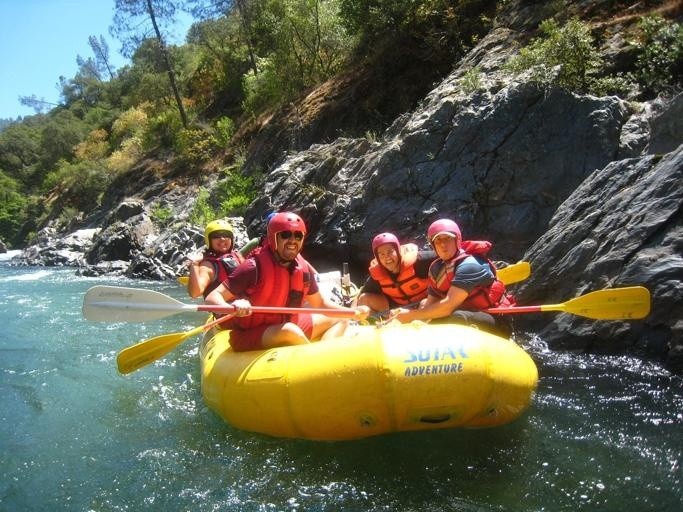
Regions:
[211,231,231,238]
[278,231,302,240]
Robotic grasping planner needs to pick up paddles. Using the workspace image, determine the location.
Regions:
[81,285,368,322]
[115,313,233,377]
[479,285,650,320]
[496,261,530,289]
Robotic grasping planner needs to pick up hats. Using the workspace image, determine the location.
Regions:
[432,231,457,243]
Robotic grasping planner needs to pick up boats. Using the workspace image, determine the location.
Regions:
[198,270,540,443]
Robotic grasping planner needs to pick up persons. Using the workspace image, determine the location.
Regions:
[397,217,517,339]
[187,217,250,305]
[204,211,373,353]
[350,231,494,312]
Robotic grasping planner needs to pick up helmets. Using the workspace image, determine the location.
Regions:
[372,233,401,268]
[427,218,461,256]
[204,220,233,250]
[267,212,305,253]
